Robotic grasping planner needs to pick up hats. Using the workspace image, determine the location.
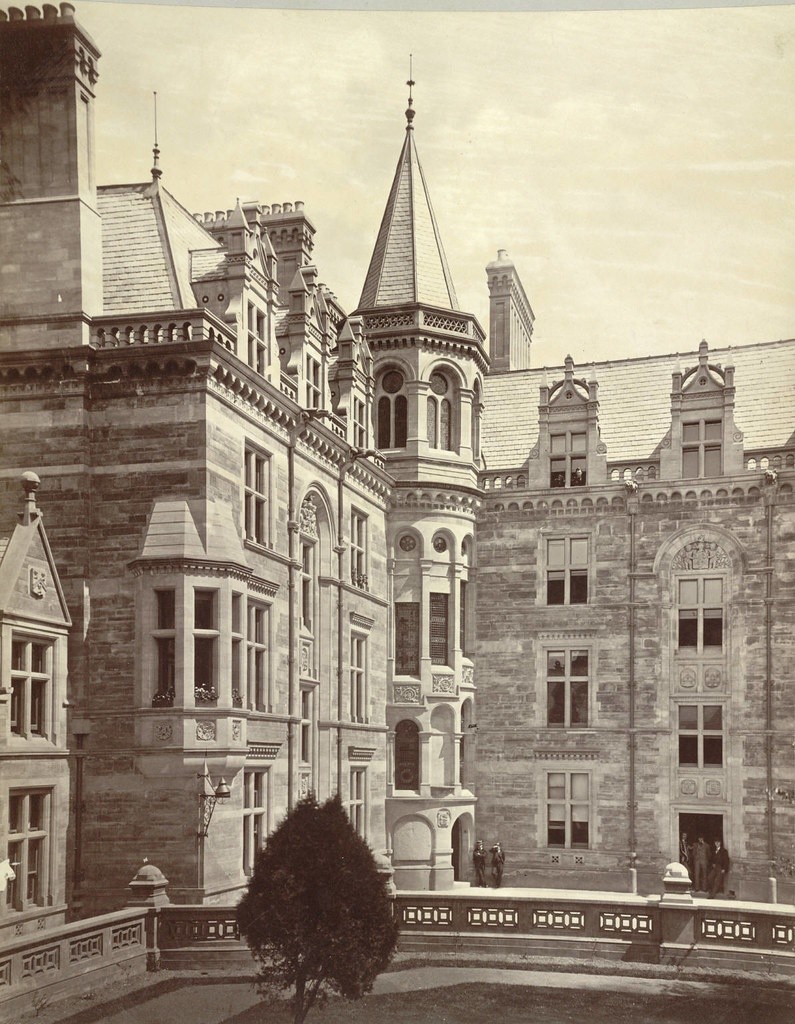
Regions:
[477,840,482,846]
[495,842,500,846]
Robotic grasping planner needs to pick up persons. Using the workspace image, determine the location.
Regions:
[489,842,505,886]
[473,840,488,888]
[680,832,692,874]
[691,834,711,892]
[706,838,728,899]
[572,466,585,486]
